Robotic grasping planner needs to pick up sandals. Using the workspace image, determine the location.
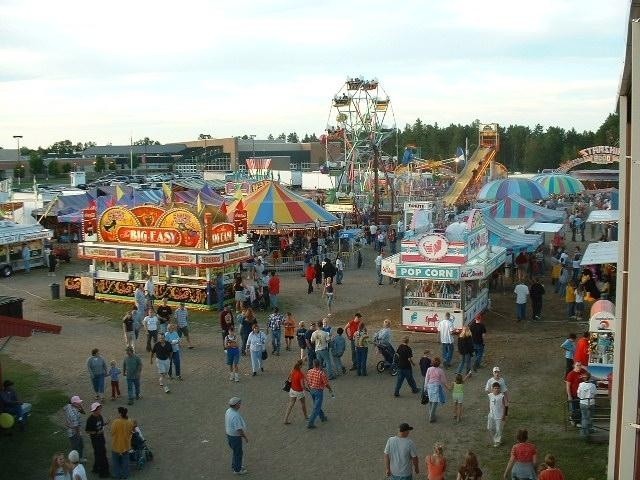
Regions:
[284,416,329,428]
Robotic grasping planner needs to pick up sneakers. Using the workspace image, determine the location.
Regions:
[158,373,185,393]
[271,347,293,357]
[230,371,239,382]
[233,465,247,475]
[94,393,143,405]
[350,363,482,424]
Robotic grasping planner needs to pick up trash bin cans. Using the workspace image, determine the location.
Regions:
[48,282,59,300]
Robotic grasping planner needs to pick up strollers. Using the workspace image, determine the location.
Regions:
[368,337,402,376]
[129,431,154,472]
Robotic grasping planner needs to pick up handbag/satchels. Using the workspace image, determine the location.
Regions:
[261,350,269,360]
[282,374,293,392]
[420,383,428,405]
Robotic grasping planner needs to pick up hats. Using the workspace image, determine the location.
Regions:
[89,402,102,412]
[493,366,501,372]
[68,449,79,463]
[399,422,413,432]
[70,396,84,404]
[229,396,242,408]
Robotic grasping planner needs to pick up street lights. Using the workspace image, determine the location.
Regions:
[250,134,257,162]
[200,133,212,149]
[13,135,24,185]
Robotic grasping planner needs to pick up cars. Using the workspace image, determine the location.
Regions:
[75,171,204,191]
[12,184,68,192]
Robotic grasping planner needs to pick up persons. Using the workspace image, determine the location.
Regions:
[478,160,483,165]
[471,168,477,175]
[327,77,376,136]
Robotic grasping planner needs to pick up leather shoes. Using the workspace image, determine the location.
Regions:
[252,368,264,377]
